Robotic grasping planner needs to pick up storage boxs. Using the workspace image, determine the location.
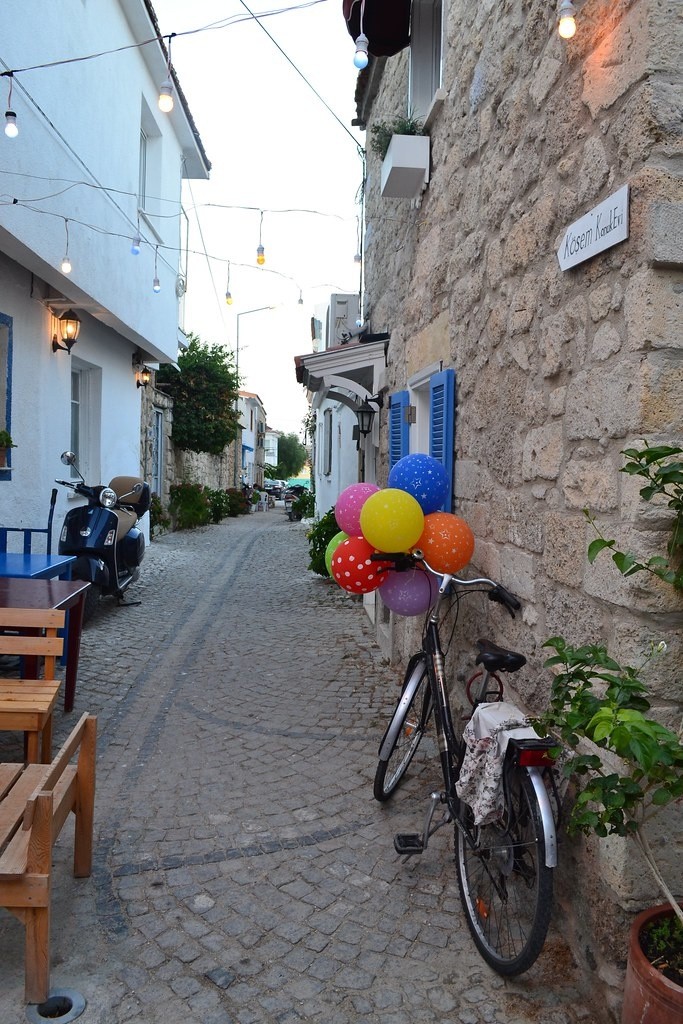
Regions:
[108,476,152,517]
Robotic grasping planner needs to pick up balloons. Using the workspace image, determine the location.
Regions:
[360,488,424,553]
[330,535,393,594]
[378,567,439,617]
[387,452,450,514]
[334,483,381,537]
[324,530,349,579]
[409,512,475,575]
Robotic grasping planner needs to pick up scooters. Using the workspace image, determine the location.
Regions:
[284,481,309,522]
[53,450,152,623]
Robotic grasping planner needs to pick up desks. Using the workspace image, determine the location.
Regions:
[0,576,92,712]
[0,552,77,678]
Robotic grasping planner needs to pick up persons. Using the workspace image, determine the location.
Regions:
[253,484,267,501]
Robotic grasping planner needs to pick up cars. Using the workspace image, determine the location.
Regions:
[263,479,285,500]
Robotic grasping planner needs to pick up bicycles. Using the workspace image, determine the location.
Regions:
[364,546,562,979]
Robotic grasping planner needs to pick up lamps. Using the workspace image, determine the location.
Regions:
[353,389,383,439]
[52,308,82,355]
[136,366,151,388]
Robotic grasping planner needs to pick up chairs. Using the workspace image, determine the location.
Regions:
[255,492,269,512]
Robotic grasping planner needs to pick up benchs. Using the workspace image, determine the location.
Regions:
[0,608,65,763]
[0,711,97,1004]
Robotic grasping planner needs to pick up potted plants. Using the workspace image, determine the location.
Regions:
[0,430,18,467]
[288,492,315,522]
[526,435,683,1024]
[149,484,259,540]
[370,106,430,210]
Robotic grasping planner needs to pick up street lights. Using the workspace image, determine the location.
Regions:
[232,305,277,489]
